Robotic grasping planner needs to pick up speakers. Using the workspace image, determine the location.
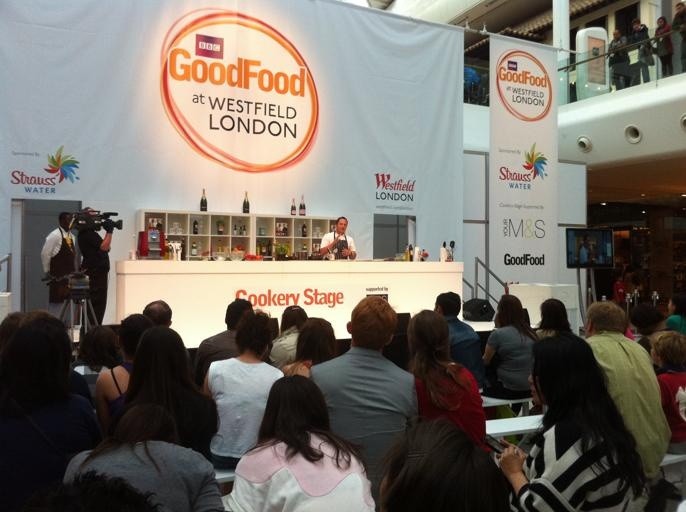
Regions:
[464,297,496,323]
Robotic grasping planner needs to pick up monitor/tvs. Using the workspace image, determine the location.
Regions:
[566,228,616,269]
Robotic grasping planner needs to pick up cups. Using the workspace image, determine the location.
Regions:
[327,253,336,261]
[312,243,320,252]
[276,222,288,236]
[169,222,185,235]
[312,227,325,238]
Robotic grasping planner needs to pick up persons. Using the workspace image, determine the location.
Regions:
[671,2,686,74]
[37,212,76,319]
[319,216,358,260]
[654,15,674,79]
[607,28,631,92]
[579,235,600,264]
[629,18,652,83]
[77,206,115,324]
[2,293,686,512]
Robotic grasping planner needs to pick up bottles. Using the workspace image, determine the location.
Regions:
[190,218,198,255]
[301,219,307,250]
[180,237,186,262]
[216,240,224,252]
[255,238,272,255]
[242,191,249,213]
[290,193,305,215]
[405,244,420,262]
[233,223,246,236]
[199,187,207,212]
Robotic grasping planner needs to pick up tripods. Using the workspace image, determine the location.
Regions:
[58,295,99,352]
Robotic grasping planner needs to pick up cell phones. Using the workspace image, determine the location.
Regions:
[483,435,508,454]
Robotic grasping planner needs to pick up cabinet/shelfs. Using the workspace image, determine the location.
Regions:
[135,208,253,260]
[253,214,338,261]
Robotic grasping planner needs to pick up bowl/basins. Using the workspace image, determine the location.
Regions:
[295,251,308,260]
[395,252,406,261]
[211,250,244,262]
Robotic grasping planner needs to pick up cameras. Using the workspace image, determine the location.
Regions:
[64,271,90,292]
